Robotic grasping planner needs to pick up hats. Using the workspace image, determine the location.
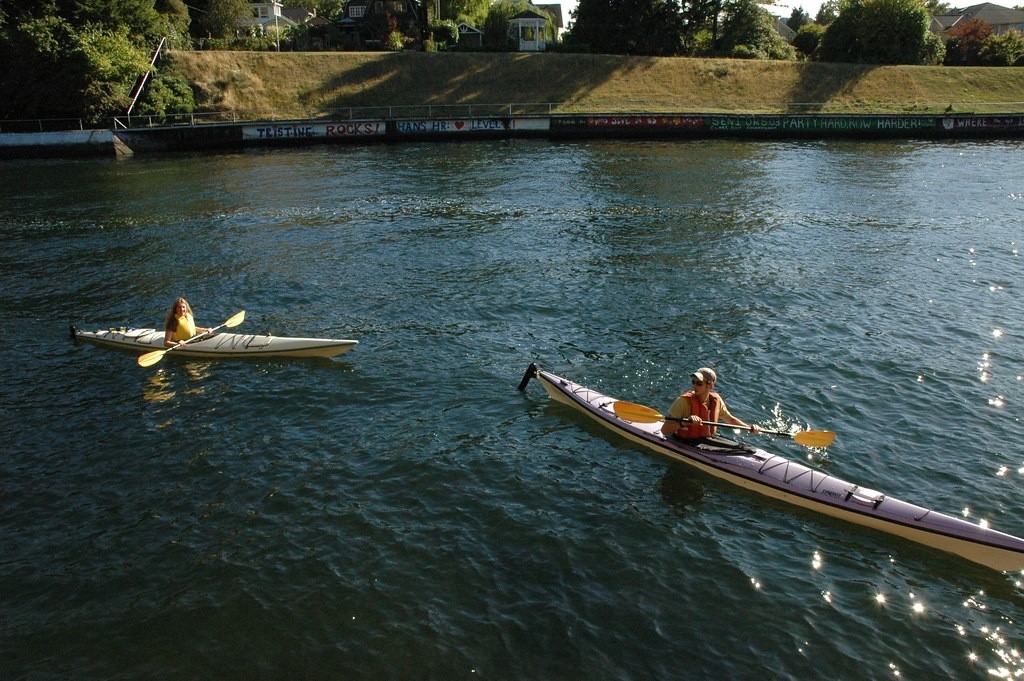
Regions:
[690,367,716,383]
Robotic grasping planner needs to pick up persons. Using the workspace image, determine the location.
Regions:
[164,296,214,348]
[659,367,762,440]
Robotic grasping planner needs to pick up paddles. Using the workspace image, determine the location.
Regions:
[612,400,836,448]
[137,310,246,368]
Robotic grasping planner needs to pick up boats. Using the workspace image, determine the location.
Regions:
[517,362,1024,573]
[70,325,359,358]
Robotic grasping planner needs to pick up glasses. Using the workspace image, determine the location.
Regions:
[692,379,708,386]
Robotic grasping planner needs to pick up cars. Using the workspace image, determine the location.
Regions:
[364,32,405,50]
